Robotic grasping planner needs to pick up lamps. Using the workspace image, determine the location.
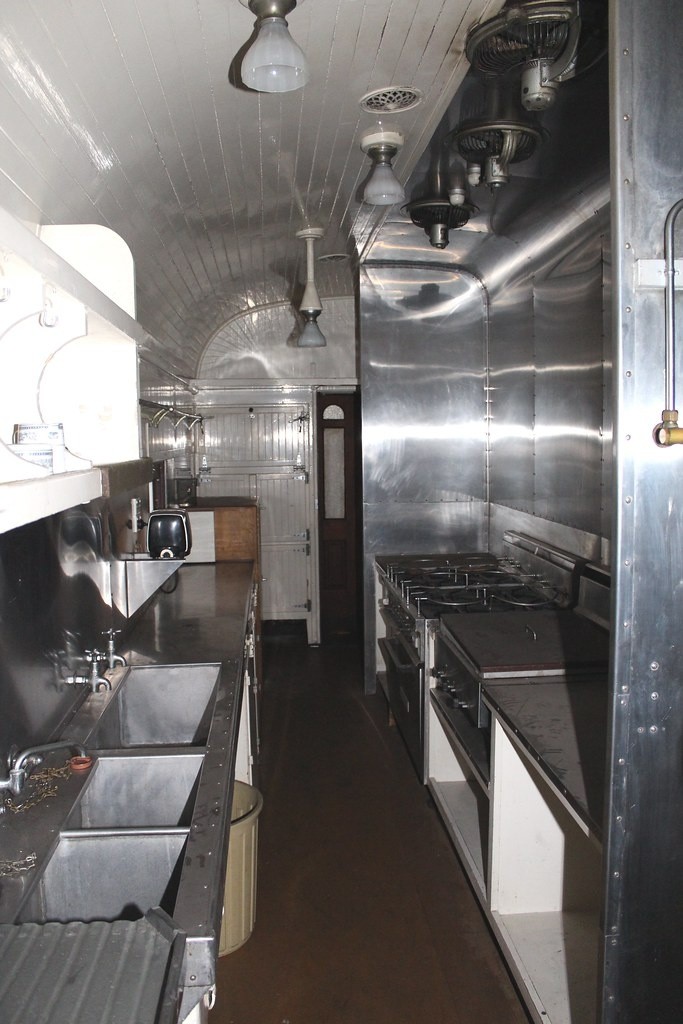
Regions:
[360,131,406,206]
[295,228,327,348]
[238,0,311,93]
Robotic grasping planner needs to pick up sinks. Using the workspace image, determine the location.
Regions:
[81,662,223,751]
[12,830,189,927]
[59,752,208,832]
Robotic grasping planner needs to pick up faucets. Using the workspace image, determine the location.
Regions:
[83,648,112,694]
[101,627,127,669]
[10,739,87,797]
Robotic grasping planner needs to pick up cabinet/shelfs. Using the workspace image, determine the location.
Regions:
[480,670,621,1023]
[196,403,321,648]
[423,610,612,909]
